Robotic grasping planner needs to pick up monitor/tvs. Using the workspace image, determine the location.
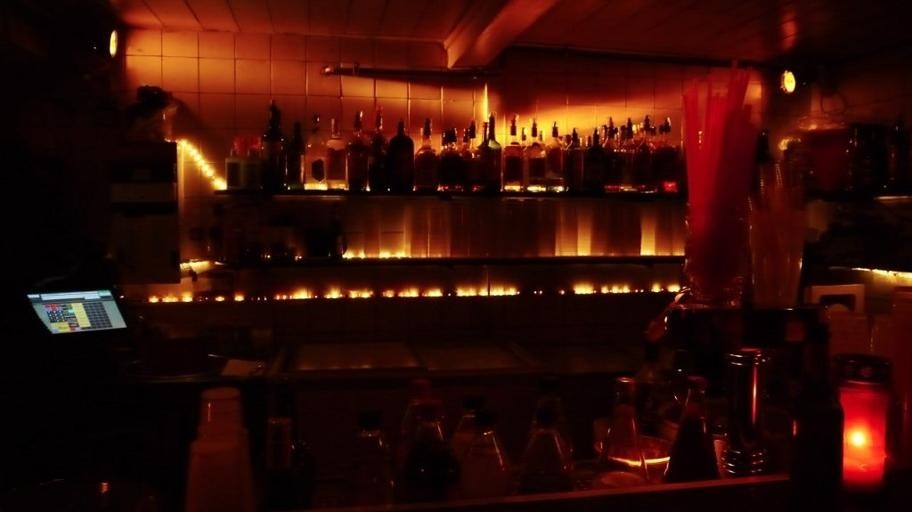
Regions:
[26,289,130,339]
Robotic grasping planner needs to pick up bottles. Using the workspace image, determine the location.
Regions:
[226,103,681,193]
[188,347,771,512]
[841,120,907,193]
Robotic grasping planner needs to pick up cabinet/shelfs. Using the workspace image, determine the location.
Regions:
[212,187,690,383]
